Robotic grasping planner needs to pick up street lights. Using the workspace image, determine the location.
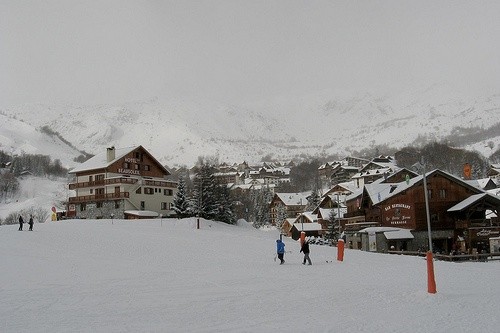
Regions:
[297,198,303,232]
[411,162,432,259]
[334,191,342,239]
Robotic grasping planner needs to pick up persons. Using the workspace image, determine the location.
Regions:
[28,218,34,231]
[19,216,24,231]
[300,240,312,265]
[276,240,285,264]
